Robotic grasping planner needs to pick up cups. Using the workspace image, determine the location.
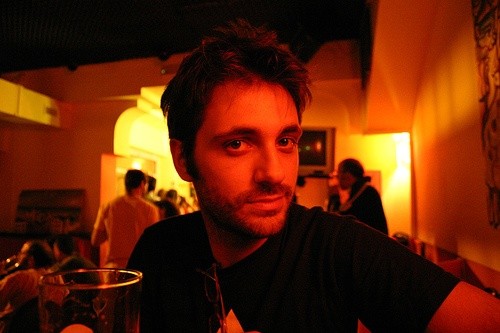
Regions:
[38,268,143,333]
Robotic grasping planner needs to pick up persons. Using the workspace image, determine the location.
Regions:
[113,22,500,333]
[1,154,390,333]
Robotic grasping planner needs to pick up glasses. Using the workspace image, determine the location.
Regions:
[195,262,228,333]
[16,251,25,259]
